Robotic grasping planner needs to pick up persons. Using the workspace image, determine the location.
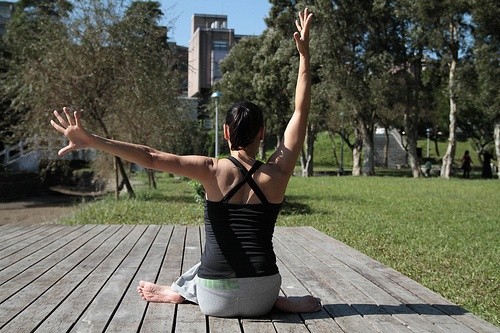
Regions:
[424,150,493,179]
[50,7,314,317]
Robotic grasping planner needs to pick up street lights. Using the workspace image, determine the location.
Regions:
[425,128,433,160]
[211,90,224,161]
